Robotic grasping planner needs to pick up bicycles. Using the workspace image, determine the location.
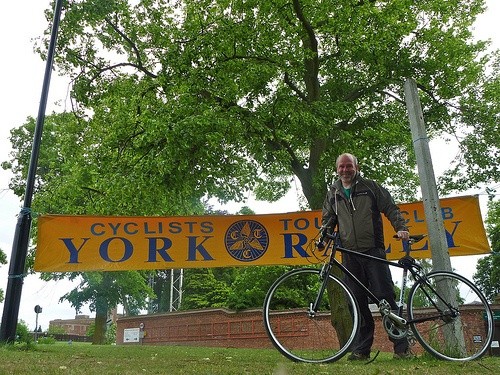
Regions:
[261,215,496,366]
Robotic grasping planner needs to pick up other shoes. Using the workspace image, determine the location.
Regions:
[347,352,372,362]
[394,350,411,359]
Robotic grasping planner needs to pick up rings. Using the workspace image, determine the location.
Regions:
[400,235,402,236]
[407,233,408,234]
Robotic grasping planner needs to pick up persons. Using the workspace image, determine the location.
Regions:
[321,153,416,360]
